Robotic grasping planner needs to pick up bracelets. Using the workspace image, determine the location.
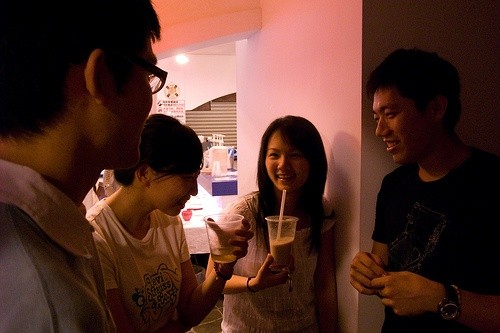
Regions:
[247,277,259,293]
[214,263,234,280]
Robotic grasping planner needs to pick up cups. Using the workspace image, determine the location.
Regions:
[266,215,298,266]
[182,210,192,221]
[203,213,244,263]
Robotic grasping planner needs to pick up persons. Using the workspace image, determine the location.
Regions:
[349,48,500,333]
[205,115,339,333]
[86,114,254,333]
[0,0,168,333]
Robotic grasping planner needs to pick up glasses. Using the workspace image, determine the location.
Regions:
[112,48,168,93]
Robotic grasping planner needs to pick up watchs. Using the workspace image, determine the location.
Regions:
[437,283,461,323]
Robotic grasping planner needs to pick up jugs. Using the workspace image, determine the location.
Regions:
[210,146,228,177]
